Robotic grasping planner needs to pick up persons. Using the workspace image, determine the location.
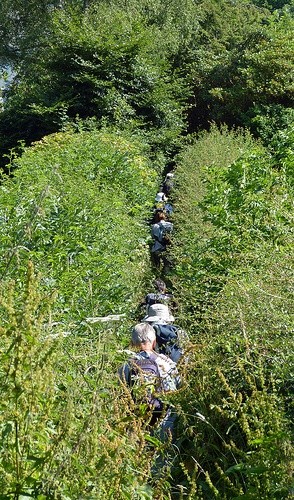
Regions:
[137,304,191,384]
[118,323,185,478]
[144,161,178,313]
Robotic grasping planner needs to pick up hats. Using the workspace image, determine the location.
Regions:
[140,303,177,322]
[155,191,168,202]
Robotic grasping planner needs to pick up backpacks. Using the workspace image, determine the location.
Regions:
[155,224,173,246]
[131,351,165,411]
[151,325,182,367]
